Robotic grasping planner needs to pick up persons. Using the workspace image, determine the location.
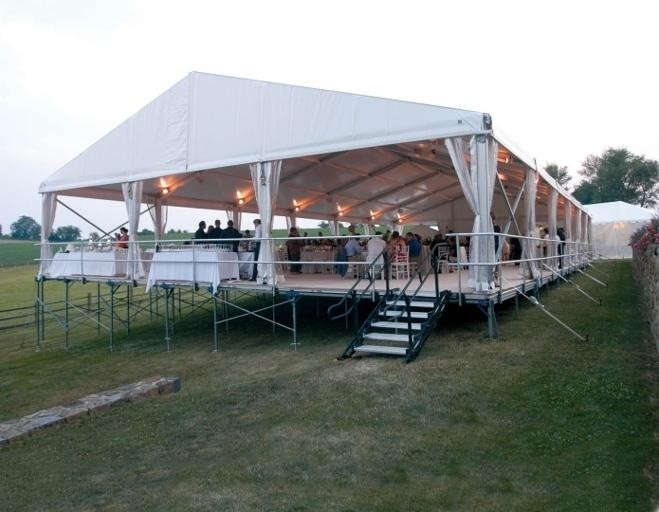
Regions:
[195,220,252,253]
[114,227,129,249]
[286,228,303,274]
[364,230,467,279]
[302,232,346,246]
[249,219,261,281]
[341,226,363,276]
[493,222,566,268]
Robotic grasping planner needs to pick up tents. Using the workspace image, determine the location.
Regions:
[579,199,657,261]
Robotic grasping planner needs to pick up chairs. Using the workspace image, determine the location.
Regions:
[277,241,510,280]
